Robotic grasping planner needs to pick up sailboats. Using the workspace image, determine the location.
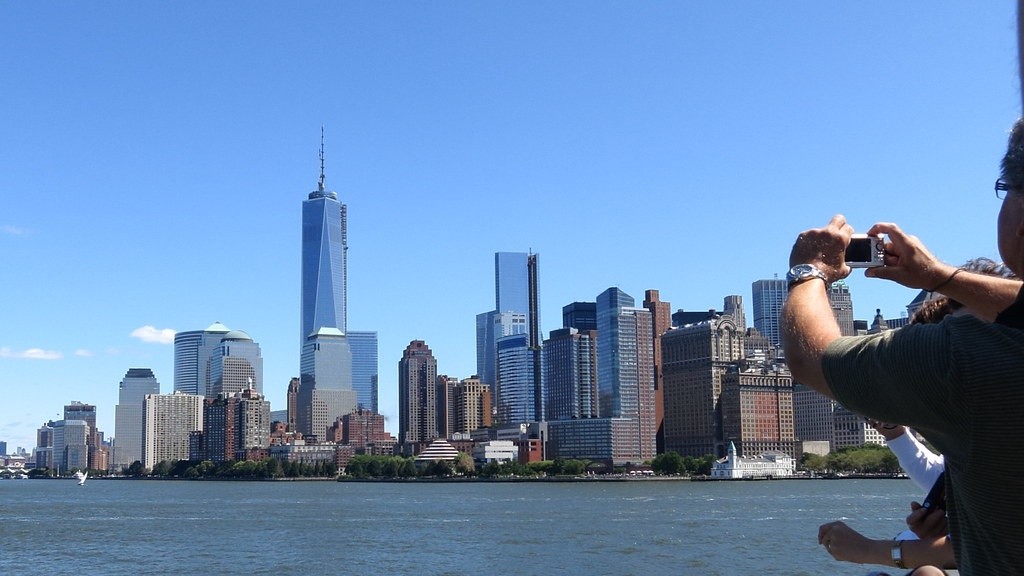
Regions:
[77,471,88,485]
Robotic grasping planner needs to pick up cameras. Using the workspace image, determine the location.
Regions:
[845,234,884,268]
[923,471,949,514]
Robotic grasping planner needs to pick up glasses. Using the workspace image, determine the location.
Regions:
[995,177,1024,200]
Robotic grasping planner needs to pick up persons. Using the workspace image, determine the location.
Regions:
[779,117,1024,576]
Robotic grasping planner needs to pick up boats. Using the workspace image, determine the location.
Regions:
[15,473,28,479]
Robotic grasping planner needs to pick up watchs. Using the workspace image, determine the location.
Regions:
[785,264,829,291]
